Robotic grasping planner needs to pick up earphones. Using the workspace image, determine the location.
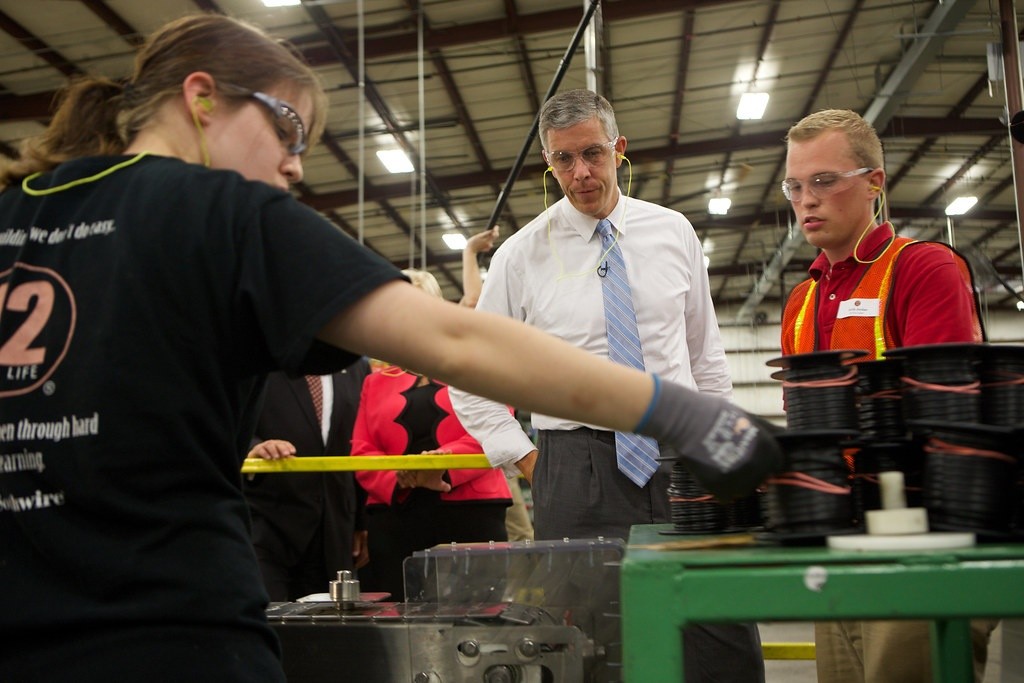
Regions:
[615,153,626,160]
[869,183,881,191]
[547,165,554,172]
[193,94,211,109]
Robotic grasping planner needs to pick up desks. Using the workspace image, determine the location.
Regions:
[618,522,1023,683]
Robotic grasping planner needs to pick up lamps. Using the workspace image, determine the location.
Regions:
[944,195,978,216]
[736,91,769,120]
[375,149,414,173]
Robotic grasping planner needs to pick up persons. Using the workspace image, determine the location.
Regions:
[452,226,536,539]
[447,89,766,683]
[345,270,512,585]
[1,13,788,682]
[781,109,1000,682]
[245,361,370,598]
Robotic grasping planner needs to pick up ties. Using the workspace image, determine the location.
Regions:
[306,373,323,427]
[595,218,662,487]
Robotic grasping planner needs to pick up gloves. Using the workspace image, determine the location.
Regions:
[634,373,784,472]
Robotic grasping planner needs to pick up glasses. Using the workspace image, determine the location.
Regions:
[546,135,619,171]
[781,167,874,202]
[215,81,307,157]
